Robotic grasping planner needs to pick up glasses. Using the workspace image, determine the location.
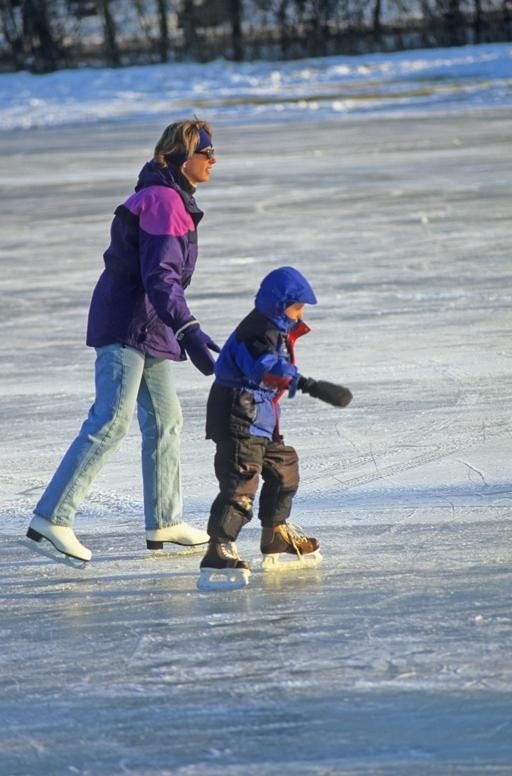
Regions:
[195,143,215,161]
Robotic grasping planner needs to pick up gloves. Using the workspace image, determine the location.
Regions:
[173,317,223,377]
[301,377,354,409]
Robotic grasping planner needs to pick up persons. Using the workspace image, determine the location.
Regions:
[200,267,351,566]
[28,121,221,563]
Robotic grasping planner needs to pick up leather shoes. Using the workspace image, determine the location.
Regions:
[146,522,210,552]
[26,514,93,563]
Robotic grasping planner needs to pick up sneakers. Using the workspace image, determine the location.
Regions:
[199,538,249,571]
[260,523,321,555]
